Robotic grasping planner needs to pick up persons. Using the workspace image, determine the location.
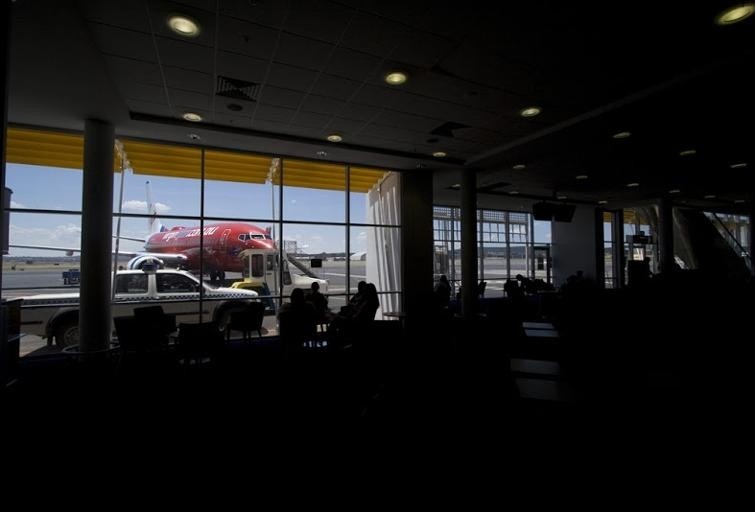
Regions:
[176,255,559,351]
[642,257,655,278]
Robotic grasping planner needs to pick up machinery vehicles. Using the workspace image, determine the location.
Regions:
[230,248,328,312]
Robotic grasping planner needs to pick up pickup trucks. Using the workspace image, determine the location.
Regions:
[6,269,262,350]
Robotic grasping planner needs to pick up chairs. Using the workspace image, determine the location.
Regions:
[113,279,487,371]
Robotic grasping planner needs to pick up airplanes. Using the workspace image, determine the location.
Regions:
[4,180,356,282]
